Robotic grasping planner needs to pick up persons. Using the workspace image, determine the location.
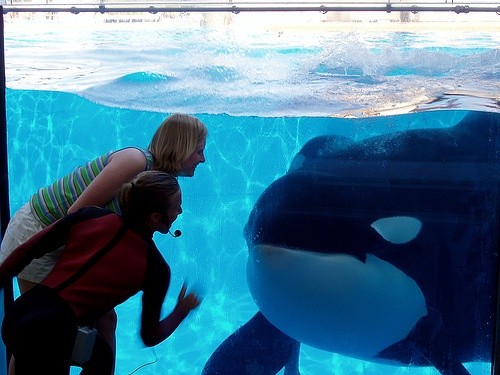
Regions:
[0,113,208,375]
[0,170,203,375]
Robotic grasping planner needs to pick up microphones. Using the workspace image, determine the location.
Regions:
[168,229,181,237]
